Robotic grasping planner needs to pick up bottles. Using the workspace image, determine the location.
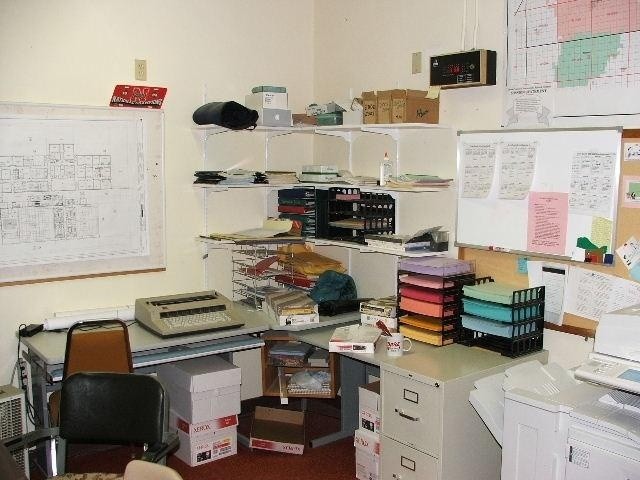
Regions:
[379,152,392,186]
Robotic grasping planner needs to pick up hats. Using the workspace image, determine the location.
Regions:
[310,269,357,304]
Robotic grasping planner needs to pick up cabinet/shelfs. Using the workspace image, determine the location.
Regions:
[199,112,453,261]
[374,368,536,480]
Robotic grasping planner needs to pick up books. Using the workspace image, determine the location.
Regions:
[363,233,431,253]
[193,171,452,185]
[268,343,329,394]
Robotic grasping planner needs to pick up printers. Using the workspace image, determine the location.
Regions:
[470,298,639,479]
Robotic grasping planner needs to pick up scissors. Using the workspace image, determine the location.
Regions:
[376,320,392,336]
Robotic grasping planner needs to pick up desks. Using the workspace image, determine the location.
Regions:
[296,329,548,480]
[23,308,268,480]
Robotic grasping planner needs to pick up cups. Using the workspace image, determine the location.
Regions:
[386,333,413,358]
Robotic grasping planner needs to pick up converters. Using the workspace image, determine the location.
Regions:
[19,324,43,337]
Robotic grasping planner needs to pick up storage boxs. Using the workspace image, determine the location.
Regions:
[361,88,441,125]
[353,382,383,480]
[152,356,239,467]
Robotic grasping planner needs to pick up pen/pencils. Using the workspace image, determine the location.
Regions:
[489,246,511,253]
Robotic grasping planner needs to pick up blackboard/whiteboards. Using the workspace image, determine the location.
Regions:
[0,101,166,287]
[454,126,624,267]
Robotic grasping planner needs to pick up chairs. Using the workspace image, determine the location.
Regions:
[2,319,177,480]
